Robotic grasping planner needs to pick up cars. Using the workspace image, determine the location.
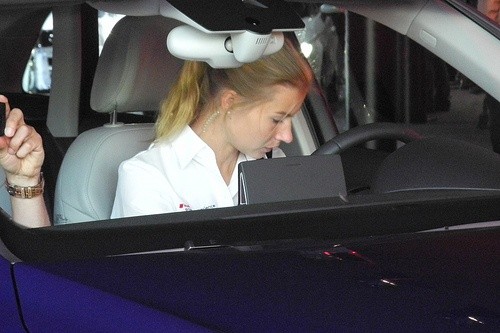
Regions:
[0,0,500,333]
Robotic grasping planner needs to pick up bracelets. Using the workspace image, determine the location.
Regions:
[5,172,45,199]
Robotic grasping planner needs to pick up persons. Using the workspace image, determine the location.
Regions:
[108,34,311,219]
[0,95,51,228]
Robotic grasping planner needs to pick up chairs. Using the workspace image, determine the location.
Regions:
[53,13,195,227]
